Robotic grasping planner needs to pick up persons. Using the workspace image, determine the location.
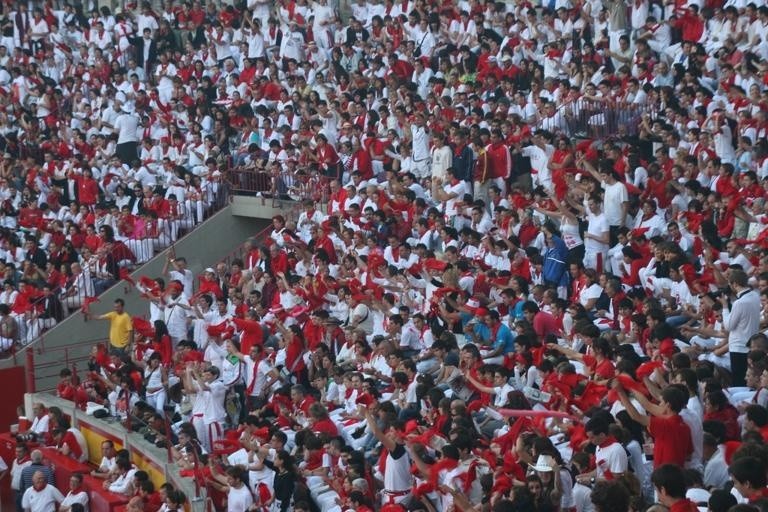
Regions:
[1,0,768,509]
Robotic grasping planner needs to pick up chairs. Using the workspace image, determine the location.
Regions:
[1,0,768,511]
